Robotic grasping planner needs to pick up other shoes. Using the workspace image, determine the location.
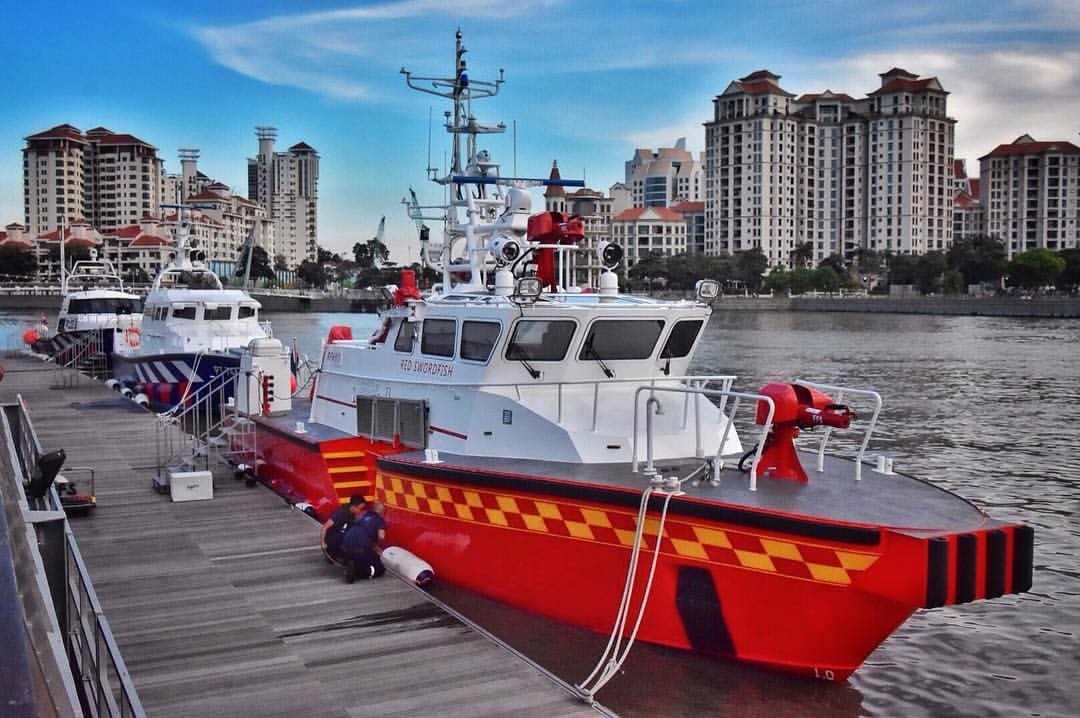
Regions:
[325,549,337,564]
[346,570,356,583]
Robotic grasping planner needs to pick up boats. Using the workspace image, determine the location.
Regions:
[226,27,1034,684]
[24,186,300,416]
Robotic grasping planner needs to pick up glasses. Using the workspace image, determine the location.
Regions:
[353,504,367,511]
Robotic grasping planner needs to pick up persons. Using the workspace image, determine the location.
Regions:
[367,317,392,350]
[39,311,47,326]
[321,492,388,584]
